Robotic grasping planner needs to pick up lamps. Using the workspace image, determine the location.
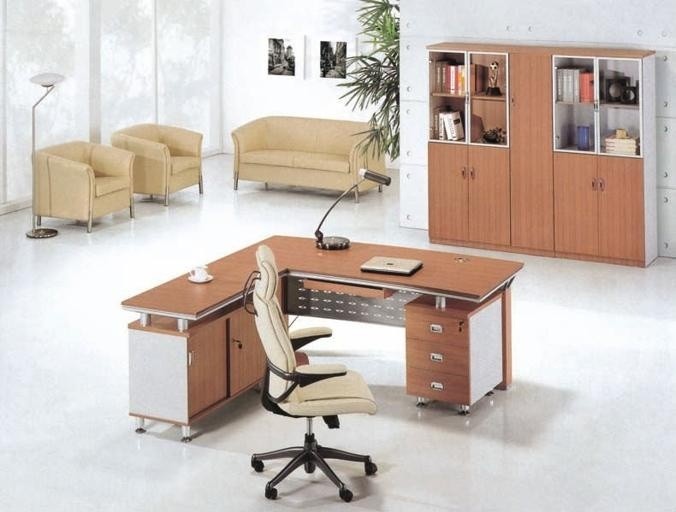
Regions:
[313,167,393,254]
[27,68,64,244]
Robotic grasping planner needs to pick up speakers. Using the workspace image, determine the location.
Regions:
[620,87,637,104]
[606,76,631,102]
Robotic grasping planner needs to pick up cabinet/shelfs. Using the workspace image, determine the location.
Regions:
[432,49,513,249]
[554,57,655,262]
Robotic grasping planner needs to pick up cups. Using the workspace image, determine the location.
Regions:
[615,129,627,139]
[577,126,591,151]
[189,265,208,280]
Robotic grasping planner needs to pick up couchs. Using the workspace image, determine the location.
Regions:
[106,121,205,206]
[29,138,134,233]
[230,116,386,200]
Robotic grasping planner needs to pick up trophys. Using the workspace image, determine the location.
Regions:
[486,61,502,96]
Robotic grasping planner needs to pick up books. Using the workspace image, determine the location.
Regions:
[471,64,486,96]
[577,124,589,151]
[554,64,595,103]
[605,134,639,155]
[430,60,465,142]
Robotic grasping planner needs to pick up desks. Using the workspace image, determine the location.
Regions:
[122,228,525,444]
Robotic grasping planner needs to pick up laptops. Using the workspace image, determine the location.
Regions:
[360,256,423,275]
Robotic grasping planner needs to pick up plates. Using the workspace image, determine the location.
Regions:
[188,275,213,283]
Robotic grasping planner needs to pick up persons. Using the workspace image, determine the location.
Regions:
[284,46,294,71]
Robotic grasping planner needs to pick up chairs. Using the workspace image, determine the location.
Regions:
[242,242,382,503]
